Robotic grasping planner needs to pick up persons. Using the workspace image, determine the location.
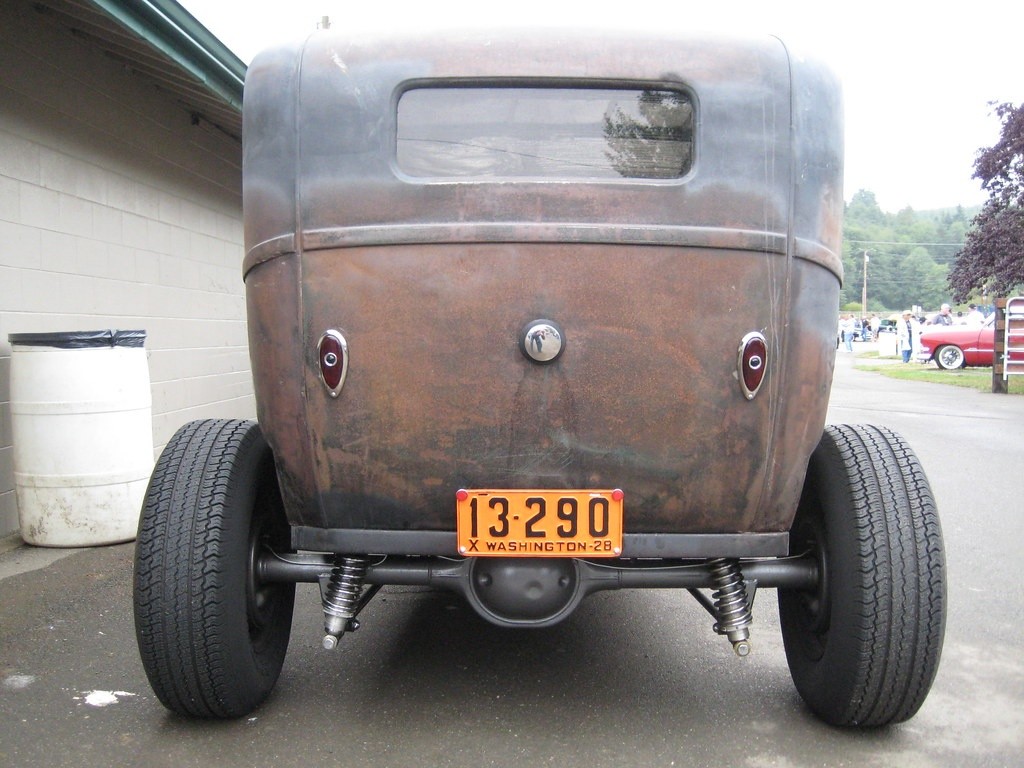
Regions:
[916,304,987,334]
[836,313,899,354]
[896,310,914,364]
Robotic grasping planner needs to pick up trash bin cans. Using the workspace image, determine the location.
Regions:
[7,330,159,545]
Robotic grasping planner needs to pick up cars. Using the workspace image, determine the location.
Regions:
[841,320,872,341]
[920,302,1024,371]
[877,319,897,334]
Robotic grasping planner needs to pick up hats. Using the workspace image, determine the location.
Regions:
[901,310,913,316]
[941,304,950,308]
[968,303,976,309]
